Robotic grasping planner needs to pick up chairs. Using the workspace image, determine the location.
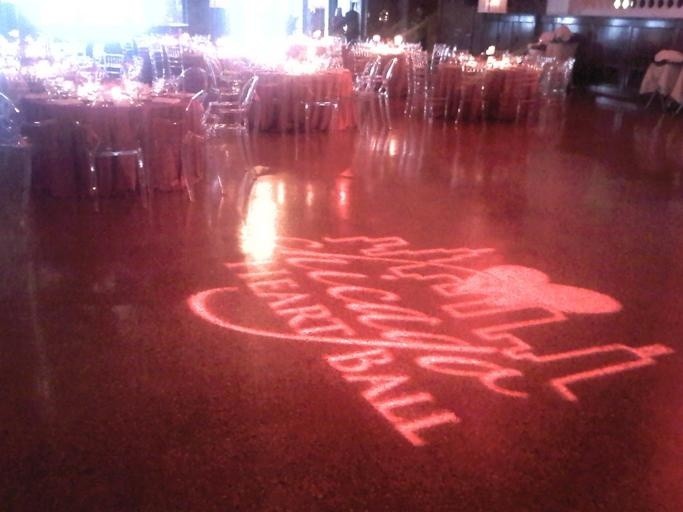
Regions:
[0,28,576,201]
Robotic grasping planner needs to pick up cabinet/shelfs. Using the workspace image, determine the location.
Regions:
[470,11,683,104]
